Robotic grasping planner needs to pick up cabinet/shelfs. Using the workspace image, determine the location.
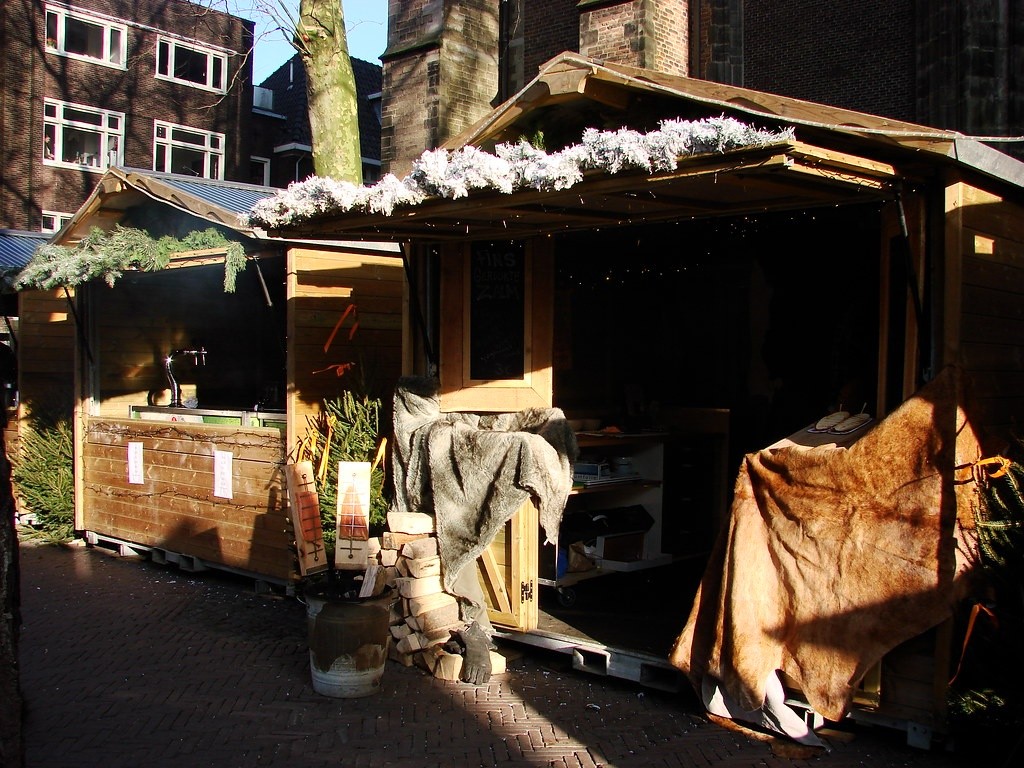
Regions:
[538,427,671,606]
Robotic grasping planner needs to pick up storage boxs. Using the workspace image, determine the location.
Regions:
[594,530,672,572]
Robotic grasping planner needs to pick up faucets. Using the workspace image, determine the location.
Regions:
[164,346,208,408]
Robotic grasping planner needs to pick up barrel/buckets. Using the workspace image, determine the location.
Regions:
[296,579,400,699]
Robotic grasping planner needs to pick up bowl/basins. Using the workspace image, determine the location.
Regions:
[581,419,601,431]
[567,419,581,432]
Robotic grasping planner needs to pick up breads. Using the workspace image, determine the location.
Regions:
[815,411,870,431]
